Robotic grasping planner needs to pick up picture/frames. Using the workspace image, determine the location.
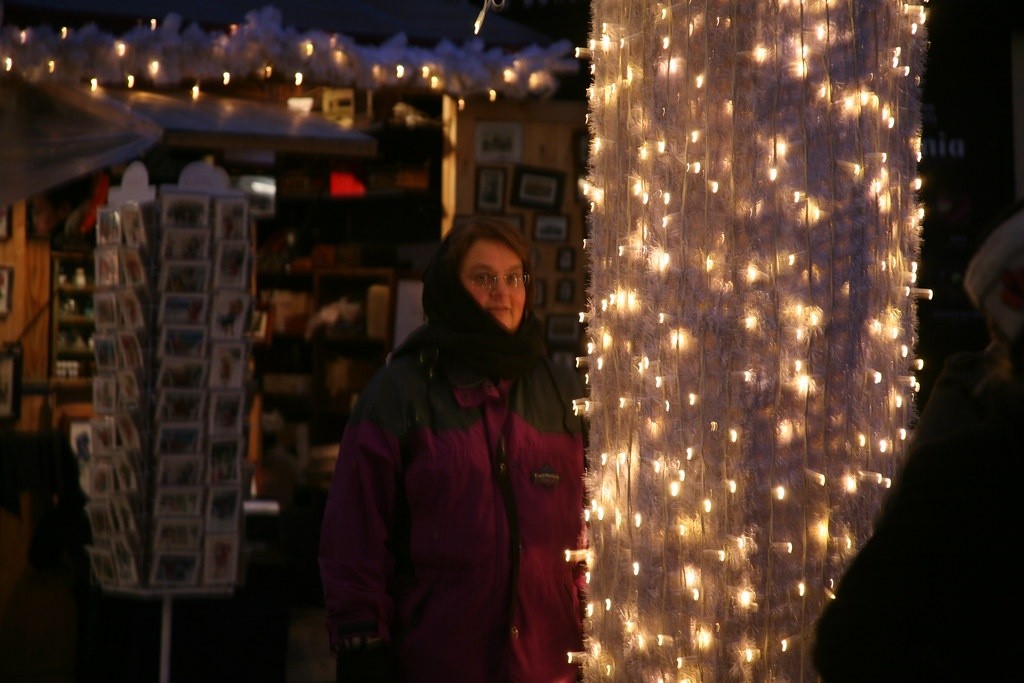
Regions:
[473,165,509,212]
[512,161,568,215]
[529,277,546,309]
[543,313,585,346]
[476,124,522,163]
[532,209,573,244]
[554,278,577,305]
[554,248,578,273]
[496,213,524,234]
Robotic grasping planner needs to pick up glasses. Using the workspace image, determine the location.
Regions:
[461,270,530,289]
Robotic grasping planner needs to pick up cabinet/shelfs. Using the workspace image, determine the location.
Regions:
[86,158,261,681]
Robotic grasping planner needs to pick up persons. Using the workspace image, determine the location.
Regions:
[313,213,586,683]
[808,213,1023,682]
